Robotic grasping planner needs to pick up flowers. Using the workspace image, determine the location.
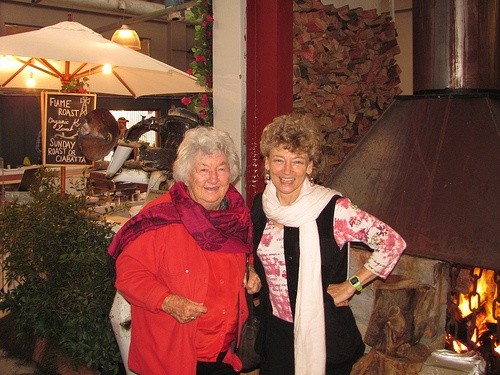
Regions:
[66,77,89,93]
[181,0,214,129]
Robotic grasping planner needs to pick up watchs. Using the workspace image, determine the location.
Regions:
[349,274,362,291]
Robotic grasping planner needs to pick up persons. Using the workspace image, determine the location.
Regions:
[113,128,263,375]
[245,114,405,375]
[114,117,134,159]
[36,130,42,165]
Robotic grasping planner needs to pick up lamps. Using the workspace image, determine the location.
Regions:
[111,10,141,50]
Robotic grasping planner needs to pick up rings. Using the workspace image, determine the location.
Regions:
[191,317,194,319]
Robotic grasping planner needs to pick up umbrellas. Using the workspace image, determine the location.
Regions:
[0,13,214,194]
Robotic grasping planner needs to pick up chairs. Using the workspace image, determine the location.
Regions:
[18,166,46,192]
